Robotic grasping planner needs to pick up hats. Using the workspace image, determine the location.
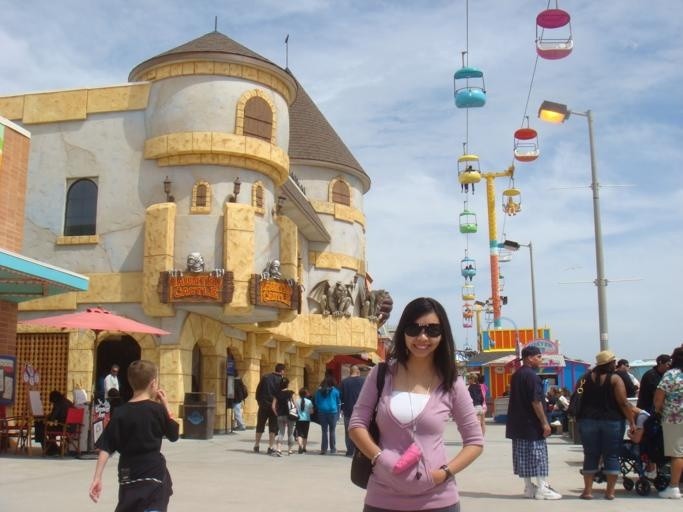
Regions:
[595,350,616,366]
[515,346,541,362]
[656,354,672,365]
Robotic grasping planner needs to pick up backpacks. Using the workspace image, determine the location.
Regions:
[616,368,635,398]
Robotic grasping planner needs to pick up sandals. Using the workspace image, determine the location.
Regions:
[580,494,593,499]
[606,493,615,500]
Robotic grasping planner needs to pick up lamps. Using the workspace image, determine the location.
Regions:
[272,196,287,216]
[230,177,241,203]
[163,176,175,202]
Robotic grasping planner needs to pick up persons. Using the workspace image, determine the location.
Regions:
[578,350,641,499]
[339,364,364,455]
[624,403,650,458]
[229,370,246,431]
[467,373,483,433]
[36,392,75,451]
[272,376,297,455]
[107,388,124,417]
[254,363,284,453]
[546,386,569,430]
[637,355,670,412]
[348,298,483,511]
[314,375,340,454]
[293,388,313,453]
[506,346,562,500]
[616,358,639,395]
[477,372,486,421]
[88,361,178,512]
[104,364,119,402]
[656,348,683,498]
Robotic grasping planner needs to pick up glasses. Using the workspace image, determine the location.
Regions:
[405,323,442,337]
[624,364,630,367]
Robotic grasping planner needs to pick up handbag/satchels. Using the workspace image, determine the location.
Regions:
[288,391,300,419]
[568,376,586,415]
[350,363,387,489]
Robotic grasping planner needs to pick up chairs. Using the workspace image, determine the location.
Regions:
[43,408,84,457]
[0,416,34,456]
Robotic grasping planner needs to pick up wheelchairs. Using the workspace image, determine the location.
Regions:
[620,439,667,496]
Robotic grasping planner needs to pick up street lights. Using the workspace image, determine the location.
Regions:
[537,101,611,353]
[499,241,538,344]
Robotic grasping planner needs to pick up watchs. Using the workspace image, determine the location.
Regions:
[439,464,455,483]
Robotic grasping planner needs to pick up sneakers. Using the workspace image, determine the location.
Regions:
[299,447,302,453]
[523,485,538,499]
[268,449,274,454]
[277,451,282,457]
[288,451,297,455]
[254,446,259,452]
[303,448,306,452]
[536,484,562,500]
[658,486,681,499]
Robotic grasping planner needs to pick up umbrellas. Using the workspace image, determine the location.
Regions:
[17,308,171,458]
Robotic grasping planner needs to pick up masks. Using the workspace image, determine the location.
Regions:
[617,359,629,367]
